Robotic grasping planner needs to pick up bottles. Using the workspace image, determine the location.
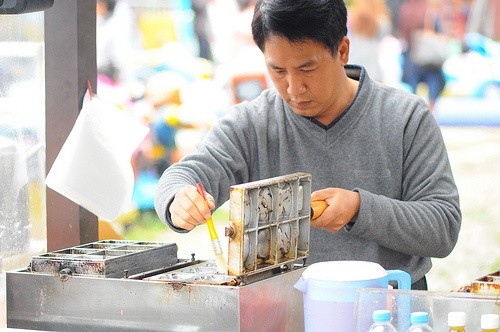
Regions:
[407,312,434,332]
[366,310,398,332]
[446,311,468,332]
[479,313,500,332]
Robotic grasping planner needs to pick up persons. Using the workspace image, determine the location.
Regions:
[401,18,446,114]
[96,0,147,109]
[154,0,461,332]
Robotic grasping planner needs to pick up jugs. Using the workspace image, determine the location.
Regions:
[292,260,411,332]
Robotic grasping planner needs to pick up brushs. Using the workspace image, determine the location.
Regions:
[196,182,227,274]
